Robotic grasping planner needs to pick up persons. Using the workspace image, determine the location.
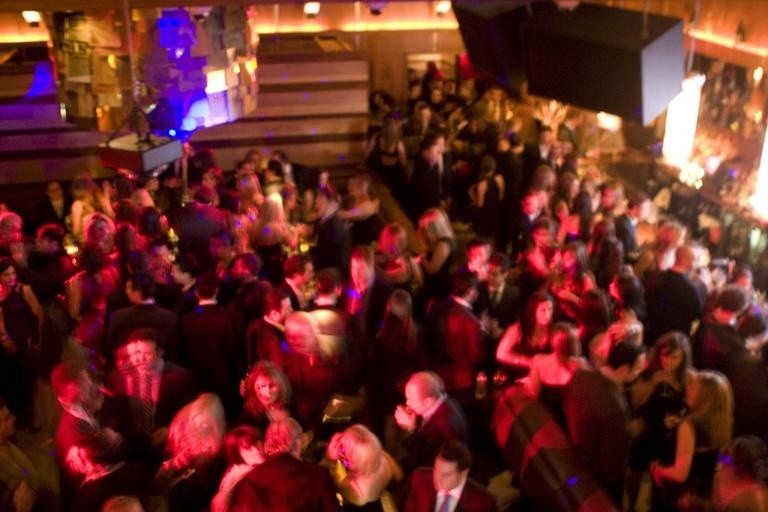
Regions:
[0,58,767,509]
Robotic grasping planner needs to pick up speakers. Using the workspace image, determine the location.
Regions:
[526,0,686,129]
[450,0,565,79]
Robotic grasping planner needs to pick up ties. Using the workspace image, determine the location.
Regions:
[143,377,153,432]
[438,494,450,512]
